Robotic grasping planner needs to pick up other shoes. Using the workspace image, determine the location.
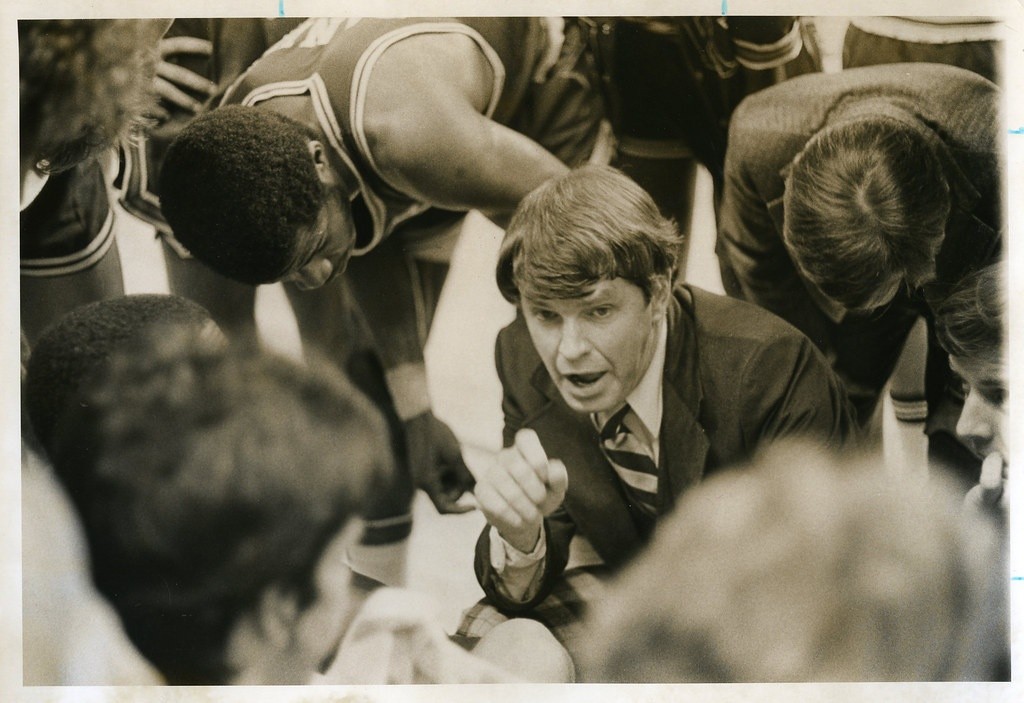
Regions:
[889,390,929,423]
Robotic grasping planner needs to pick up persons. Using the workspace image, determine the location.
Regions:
[21,16,1010,684]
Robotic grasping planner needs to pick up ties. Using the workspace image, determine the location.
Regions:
[595,401,660,522]
